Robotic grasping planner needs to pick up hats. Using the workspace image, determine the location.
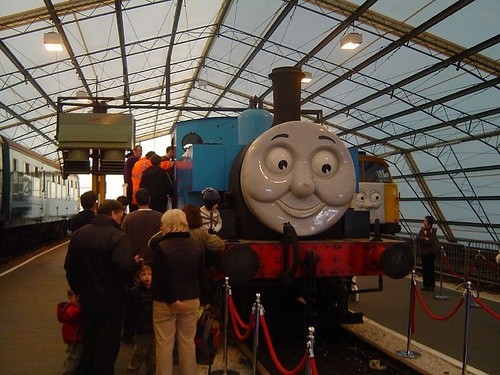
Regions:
[202,187,219,211]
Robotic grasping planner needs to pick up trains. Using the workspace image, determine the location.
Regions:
[166,67,415,324]
[0,134,81,253]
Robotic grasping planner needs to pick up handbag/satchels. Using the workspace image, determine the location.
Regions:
[195,308,220,366]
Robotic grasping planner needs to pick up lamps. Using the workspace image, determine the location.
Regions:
[339,20,362,50]
[301,65,312,84]
[43,25,64,52]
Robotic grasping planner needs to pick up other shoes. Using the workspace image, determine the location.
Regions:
[421,287,427,291]
[429,287,434,292]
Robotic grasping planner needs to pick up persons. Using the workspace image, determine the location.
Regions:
[415,216,437,292]
[68,145,223,346]
[57,286,84,375]
[149,209,213,375]
[126,261,156,375]
[64,200,144,375]
